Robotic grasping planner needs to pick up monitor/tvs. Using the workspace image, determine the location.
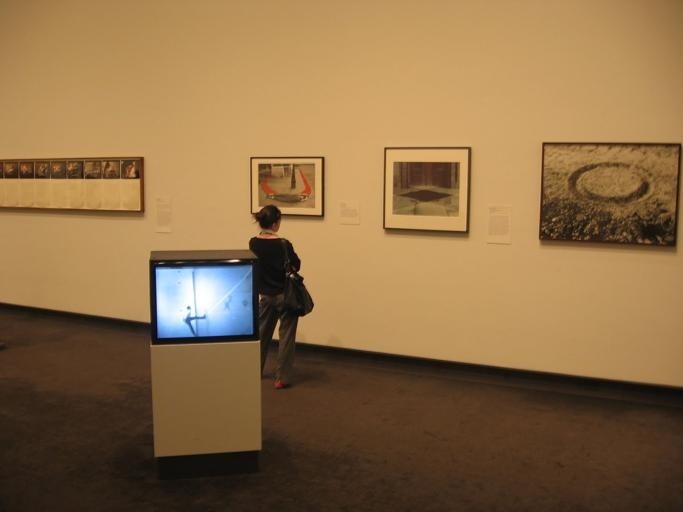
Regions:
[149,249,259,343]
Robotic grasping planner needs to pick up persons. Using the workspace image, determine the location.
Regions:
[249,205,301,390]
[183,306,206,337]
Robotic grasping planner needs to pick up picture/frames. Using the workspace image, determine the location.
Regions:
[0,158,144,213]
[251,157,324,217]
[540,142,681,246]
[383,148,471,233]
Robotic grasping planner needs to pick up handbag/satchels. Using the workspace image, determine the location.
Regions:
[280,262,315,317]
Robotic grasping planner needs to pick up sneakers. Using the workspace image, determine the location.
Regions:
[273,377,286,389]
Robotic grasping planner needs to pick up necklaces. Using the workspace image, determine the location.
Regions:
[258,229,275,235]
